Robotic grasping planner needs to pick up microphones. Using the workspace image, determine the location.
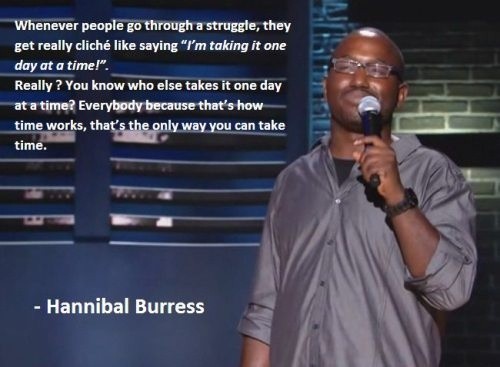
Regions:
[358,95,384,189]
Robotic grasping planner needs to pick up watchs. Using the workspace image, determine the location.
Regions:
[386,187,418,220]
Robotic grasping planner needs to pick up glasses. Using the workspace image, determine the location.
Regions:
[327,57,403,80]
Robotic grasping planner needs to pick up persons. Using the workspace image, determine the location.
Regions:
[237,27,477,367]
